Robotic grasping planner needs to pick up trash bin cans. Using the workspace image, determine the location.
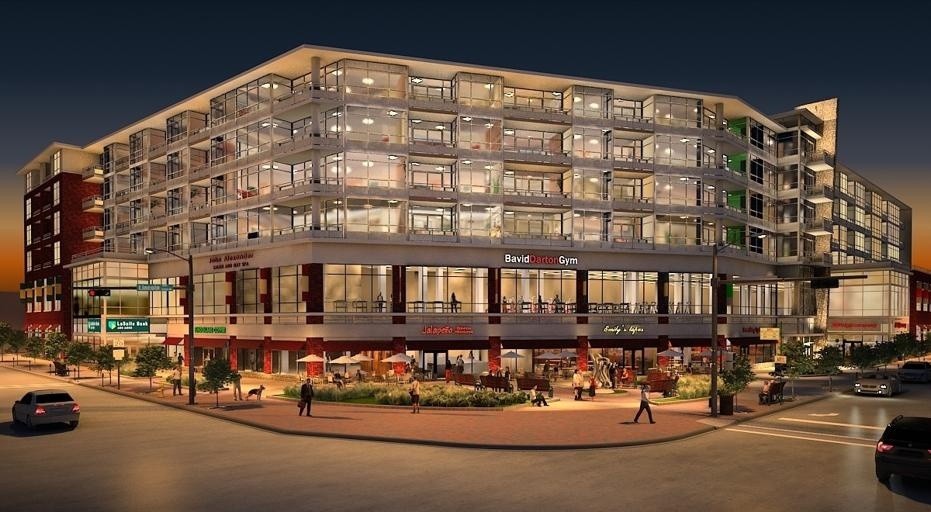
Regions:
[720,392,734,415]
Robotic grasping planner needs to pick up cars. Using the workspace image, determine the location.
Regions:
[11,389,80,430]
[853,362,931,397]
[874,414,931,483]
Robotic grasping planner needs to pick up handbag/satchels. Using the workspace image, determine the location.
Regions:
[408,391,413,396]
[297,401,306,408]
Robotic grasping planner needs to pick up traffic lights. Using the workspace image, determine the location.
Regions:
[88,289,110,296]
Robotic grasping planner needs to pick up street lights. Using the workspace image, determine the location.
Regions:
[711,233,768,418]
[145,247,194,404]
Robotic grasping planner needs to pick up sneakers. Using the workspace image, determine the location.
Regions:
[634,419,656,424]
[537,403,549,407]
[410,411,420,414]
[298,413,312,417]
[575,396,595,401]
